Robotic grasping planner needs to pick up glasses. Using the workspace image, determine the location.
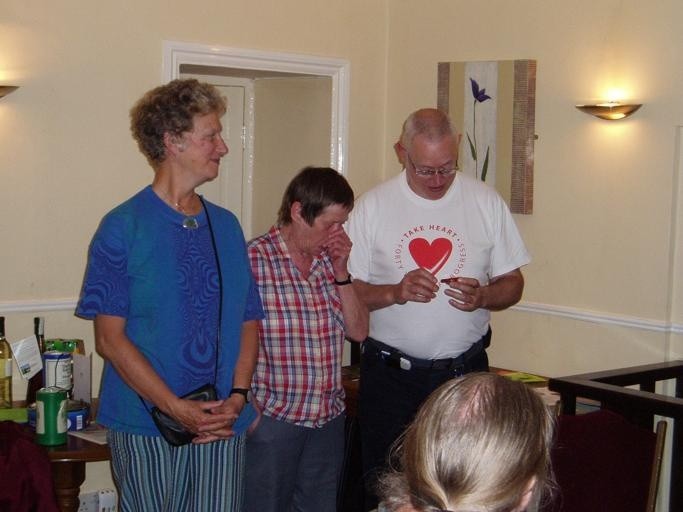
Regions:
[407,153,459,177]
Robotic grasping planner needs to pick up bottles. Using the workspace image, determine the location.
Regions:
[27,316,43,406]
[44,348,74,398]
[0,317,12,409]
[35,391,68,446]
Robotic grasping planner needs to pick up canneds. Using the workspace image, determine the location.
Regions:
[28,386,89,446]
[43,352,73,391]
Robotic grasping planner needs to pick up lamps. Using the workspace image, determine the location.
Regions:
[0,86,19,98]
[575,100,643,120]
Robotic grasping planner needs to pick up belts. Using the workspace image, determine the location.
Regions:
[397,341,485,371]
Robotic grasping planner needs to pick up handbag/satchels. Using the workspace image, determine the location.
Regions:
[150,384,218,446]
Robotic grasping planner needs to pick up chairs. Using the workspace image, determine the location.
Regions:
[540,404,667,512]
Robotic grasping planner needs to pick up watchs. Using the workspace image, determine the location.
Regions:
[333,272,356,287]
[228,387,253,404]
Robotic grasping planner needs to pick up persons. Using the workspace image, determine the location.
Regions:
[71,76,263,512]
[337,106,534,512]
[368,370,559,512]
[241,165,371,512]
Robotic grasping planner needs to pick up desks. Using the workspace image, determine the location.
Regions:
[340,366,560,511]
[11,398,112,512]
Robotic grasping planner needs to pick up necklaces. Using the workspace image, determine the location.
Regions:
[154,180,200,230]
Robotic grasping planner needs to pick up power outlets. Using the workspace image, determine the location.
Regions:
[78,492,99,512]
